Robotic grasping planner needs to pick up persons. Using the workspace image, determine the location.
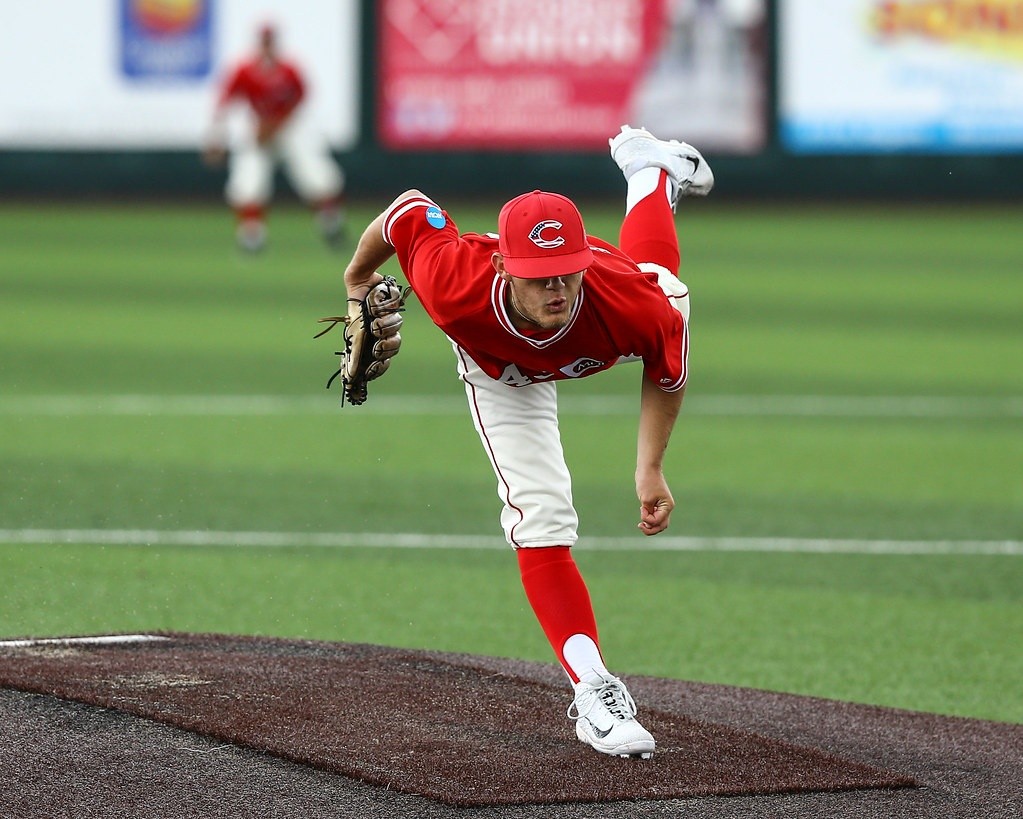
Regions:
[211,26,342,253]
[345,125,714,760]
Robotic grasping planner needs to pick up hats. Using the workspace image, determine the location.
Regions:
[498,190,594,279]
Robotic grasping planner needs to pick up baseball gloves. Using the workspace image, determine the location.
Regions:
[312,272,413,409]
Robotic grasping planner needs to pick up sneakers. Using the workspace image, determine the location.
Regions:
[608,124,715,214]
[566,668,657,759]
